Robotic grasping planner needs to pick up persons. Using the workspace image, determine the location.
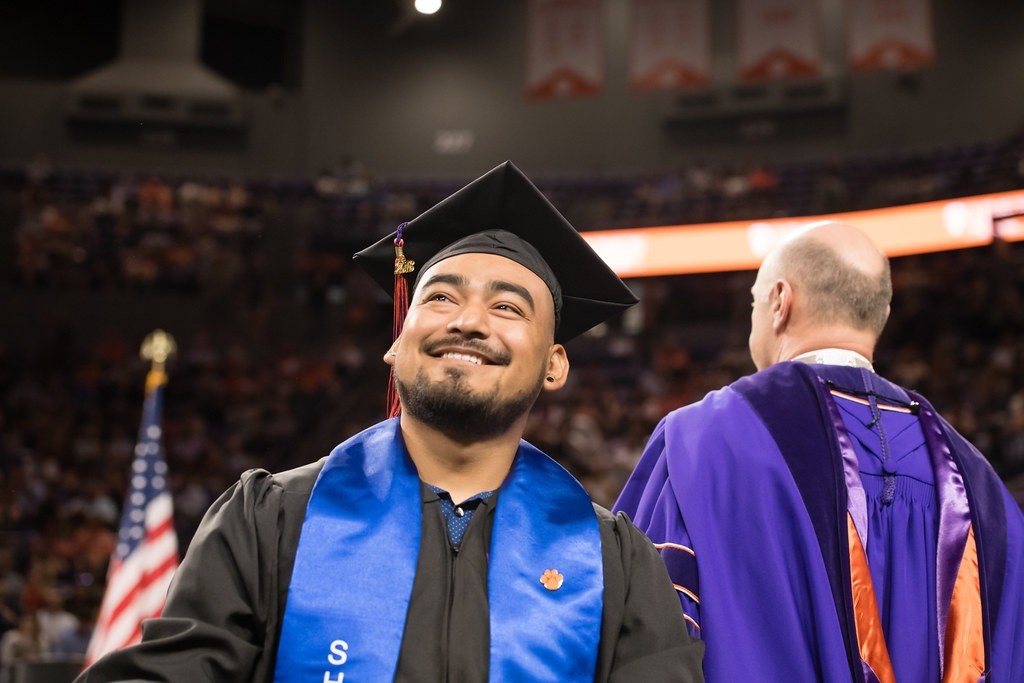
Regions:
[612,220,1024,683]
[71,159,706,682]
[1,134,1024,683]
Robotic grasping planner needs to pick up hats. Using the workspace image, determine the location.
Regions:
[353,158,639,419]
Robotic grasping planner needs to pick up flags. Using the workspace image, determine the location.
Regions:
[81,374,179,674]
